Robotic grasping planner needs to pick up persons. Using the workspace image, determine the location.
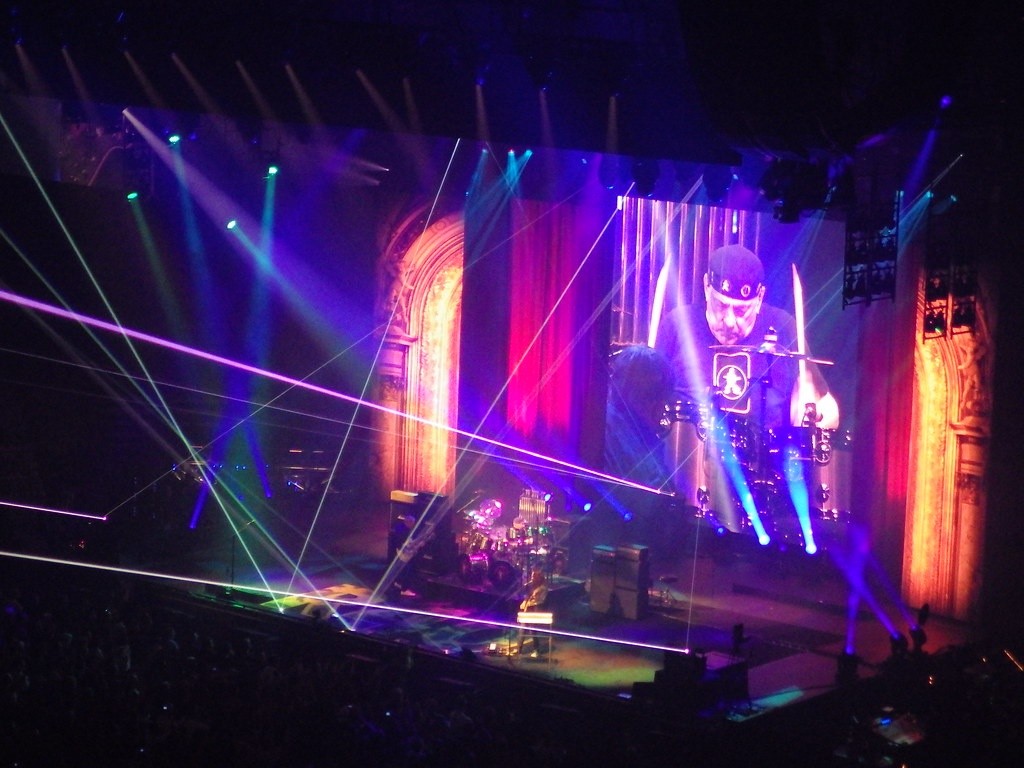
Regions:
[602,344,688,513]
[514,566,549,658]
[647,244,839,539]
[0,580,676,768]
[384,514,417,603]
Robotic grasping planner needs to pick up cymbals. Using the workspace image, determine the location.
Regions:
[707,341,834,365]
[463,508,494,526]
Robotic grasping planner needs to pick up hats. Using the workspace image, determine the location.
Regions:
[613,346,674,403]
[708,244,765,300]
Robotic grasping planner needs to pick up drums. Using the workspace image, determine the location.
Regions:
[458,526,548,589]
[706,407,813,521]
